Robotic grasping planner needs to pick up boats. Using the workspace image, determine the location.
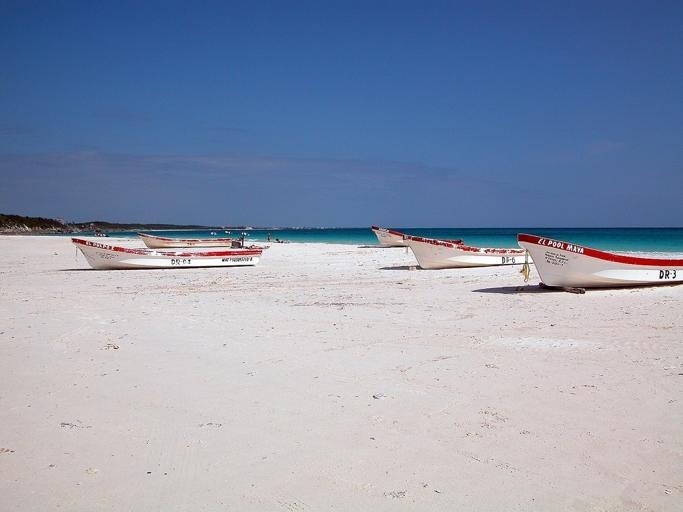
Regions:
[515,232,682,287]
[401,232,534,269]
[369,223,465,250]
[135,231,235,249]
[70,235,263,271]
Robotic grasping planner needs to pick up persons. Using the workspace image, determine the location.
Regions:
[266,232,271,242]
